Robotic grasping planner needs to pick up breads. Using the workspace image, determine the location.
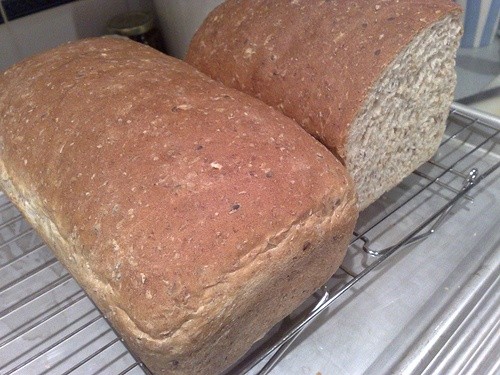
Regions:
[0,33,361,375]
[185,0,464,211]
